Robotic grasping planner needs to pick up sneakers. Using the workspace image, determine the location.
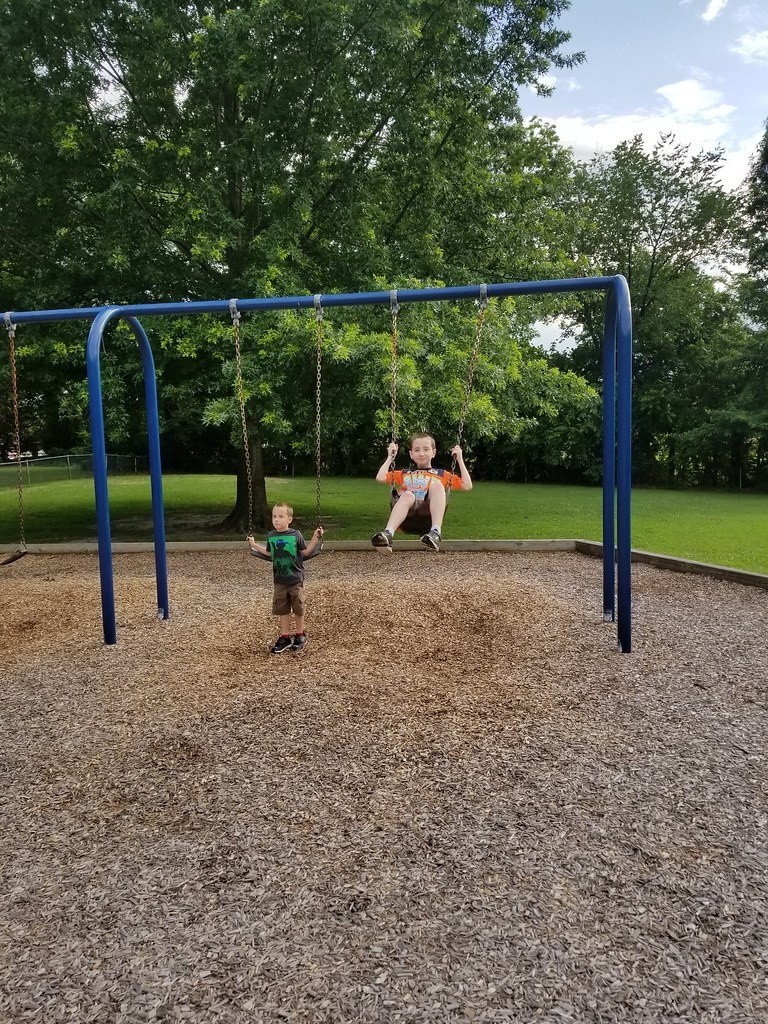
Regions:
[372,530,392,556]
[420,529,441,552]
[292,633,307,651]
[271,637,293,653]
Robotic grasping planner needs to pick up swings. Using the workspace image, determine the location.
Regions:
[233,314,326,561]
[0,331,28,567]
[390,302,488,535]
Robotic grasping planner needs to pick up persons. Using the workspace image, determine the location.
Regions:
[371,432,473,555]
[246,502,324,653]
